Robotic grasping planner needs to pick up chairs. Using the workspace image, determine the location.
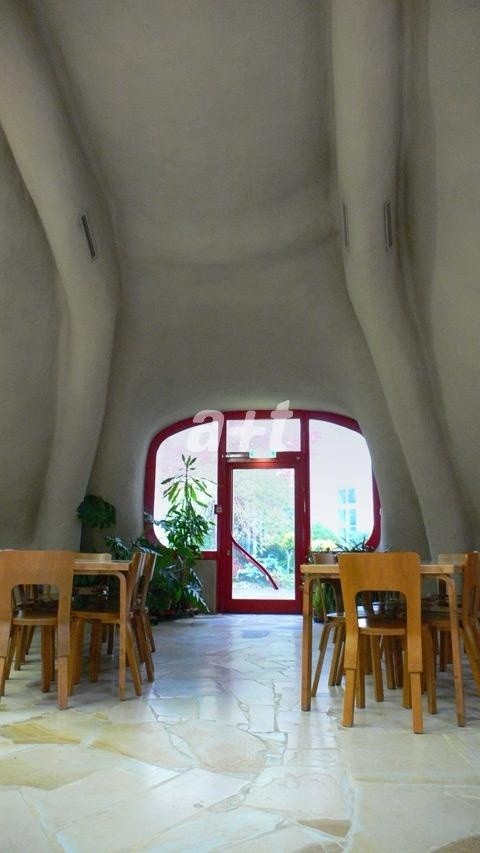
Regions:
[297,547,480,736]
[0,546,160,711]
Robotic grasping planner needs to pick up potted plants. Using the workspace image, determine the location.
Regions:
[75,454,218,618]
